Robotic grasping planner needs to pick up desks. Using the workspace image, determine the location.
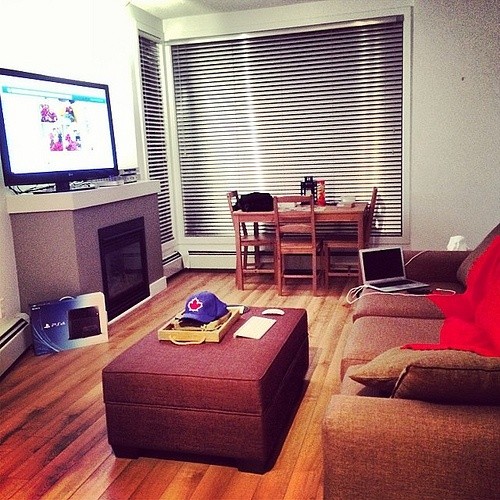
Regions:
[234,202,368,285]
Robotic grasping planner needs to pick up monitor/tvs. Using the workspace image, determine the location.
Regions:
[0,68,119,195]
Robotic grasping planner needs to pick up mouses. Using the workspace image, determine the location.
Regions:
[261,309,285,316]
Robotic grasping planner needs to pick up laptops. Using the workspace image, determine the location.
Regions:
[359,246,430,293]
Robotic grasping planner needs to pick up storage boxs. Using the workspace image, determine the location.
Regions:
[158,307,240,343]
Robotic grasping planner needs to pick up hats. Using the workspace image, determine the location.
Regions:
[178,291,229,323]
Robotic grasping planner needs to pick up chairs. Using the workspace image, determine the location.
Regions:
[321,186,377,286]
[272,196,325,292]
[227,190,277,287]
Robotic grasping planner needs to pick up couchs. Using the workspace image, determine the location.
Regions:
[322,223,500,500]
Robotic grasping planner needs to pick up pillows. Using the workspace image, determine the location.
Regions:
[348,346,461,390]
[389,354,500,405]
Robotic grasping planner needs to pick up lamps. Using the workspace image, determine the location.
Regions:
[301,175,317,206]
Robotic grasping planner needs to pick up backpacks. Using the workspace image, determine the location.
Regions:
[232,192,274,212]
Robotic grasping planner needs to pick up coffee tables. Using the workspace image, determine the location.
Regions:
[101,306,308,475]
